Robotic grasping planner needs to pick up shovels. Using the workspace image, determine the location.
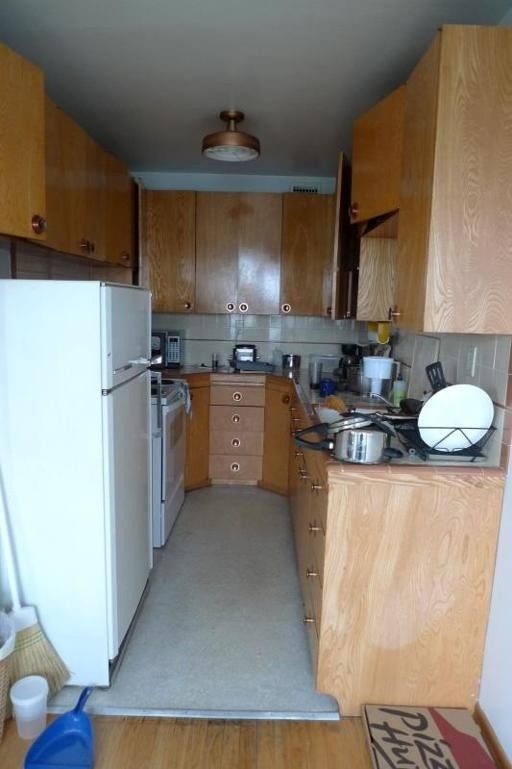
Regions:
[425,361,447,393]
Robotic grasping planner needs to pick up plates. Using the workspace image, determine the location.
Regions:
[418,384,494,452]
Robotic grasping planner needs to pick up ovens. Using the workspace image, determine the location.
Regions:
[161,390,185,499]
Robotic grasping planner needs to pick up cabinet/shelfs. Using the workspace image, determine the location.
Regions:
[109,154,134,267]
[265,375,295,494]
[390,27,512,334]
[196,187,281,314]
[209,374,265,485]
[282,191,335,314]
[45,93,109,264]
[184,374,210,490]
[1,37,46,250]
[292,399,506,715]
[330,82,407,323]
[140,189,196,313]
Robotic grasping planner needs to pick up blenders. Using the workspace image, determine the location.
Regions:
[363,356,400,407]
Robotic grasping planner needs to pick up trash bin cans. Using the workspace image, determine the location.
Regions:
[0,611,16,743]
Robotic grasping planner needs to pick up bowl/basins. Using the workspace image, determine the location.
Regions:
[385,405,404,414]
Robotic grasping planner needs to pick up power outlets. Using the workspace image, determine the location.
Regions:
[235,319,244,337]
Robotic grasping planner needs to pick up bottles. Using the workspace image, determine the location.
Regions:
[319,378,335,397]
[394,373,408,407]
[273,347,282,367]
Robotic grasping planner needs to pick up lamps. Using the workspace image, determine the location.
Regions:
[201,107,262,165]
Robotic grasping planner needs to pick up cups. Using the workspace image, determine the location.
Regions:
[212,352,219,370]
[9,675,49,740]
[308,363,321,390]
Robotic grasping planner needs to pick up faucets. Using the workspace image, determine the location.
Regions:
[371,393,400,407]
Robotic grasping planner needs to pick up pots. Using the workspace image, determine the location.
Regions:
[294,414,402,464]
[282,352,301,370]
[286,371,302,387]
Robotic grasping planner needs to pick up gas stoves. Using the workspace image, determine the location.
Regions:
[151,375,185,405]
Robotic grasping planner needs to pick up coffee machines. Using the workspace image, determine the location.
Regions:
[335,344,362,391]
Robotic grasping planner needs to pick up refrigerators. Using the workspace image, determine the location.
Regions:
[1,279,153,667]
